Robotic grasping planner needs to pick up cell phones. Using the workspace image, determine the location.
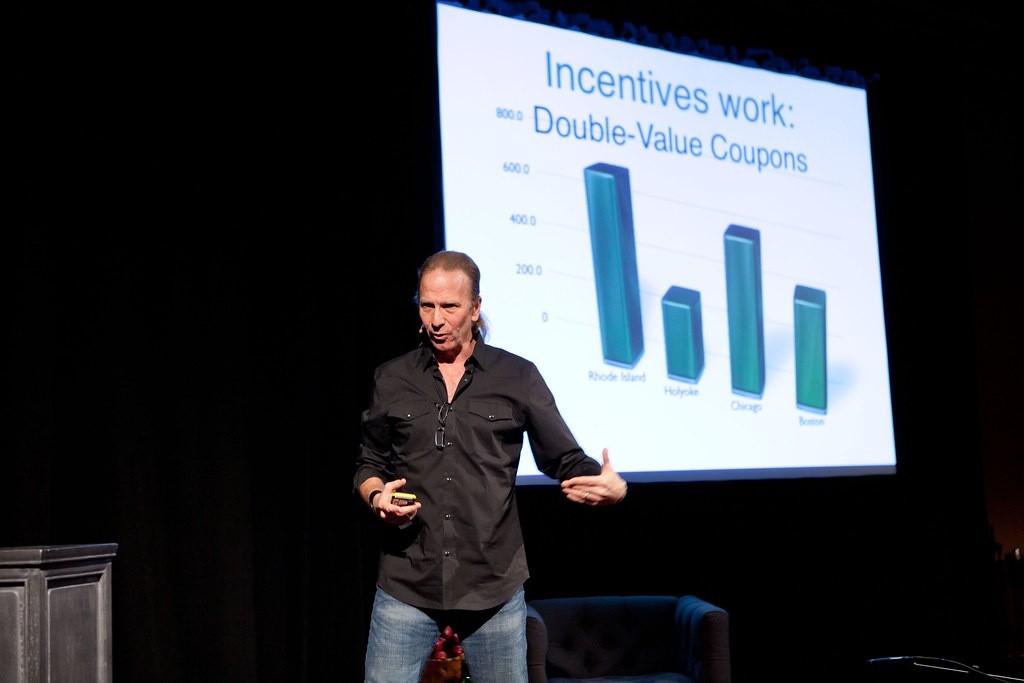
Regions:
[389,492,416,507]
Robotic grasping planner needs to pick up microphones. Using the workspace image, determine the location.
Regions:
[419,325,424,333]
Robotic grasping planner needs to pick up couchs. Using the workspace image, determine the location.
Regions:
[526,595,734,683]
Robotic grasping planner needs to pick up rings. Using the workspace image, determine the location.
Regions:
[585,493,589,501]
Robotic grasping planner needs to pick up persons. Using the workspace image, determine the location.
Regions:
[354,251,629,683]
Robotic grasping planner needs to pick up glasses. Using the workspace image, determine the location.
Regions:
[434,402,450,449]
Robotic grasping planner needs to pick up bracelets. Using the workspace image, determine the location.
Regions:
[369,490,382,510]
